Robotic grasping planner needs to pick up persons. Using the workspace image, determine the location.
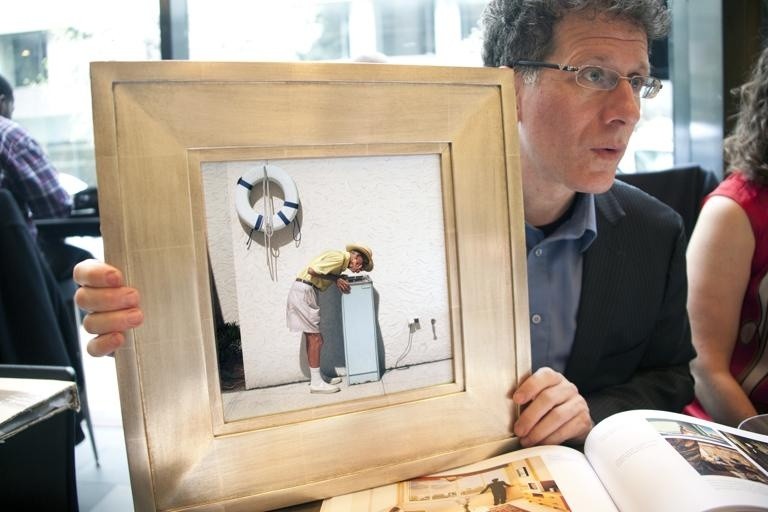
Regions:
[65,0,702,459]
[284,243,374,396]
[0,75,99,331]
[682,46,767,441]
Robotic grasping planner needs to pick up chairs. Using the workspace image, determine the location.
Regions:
[618,167,724,241]
[0,164,84,383]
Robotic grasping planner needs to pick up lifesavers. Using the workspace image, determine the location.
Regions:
[236,165,299,233]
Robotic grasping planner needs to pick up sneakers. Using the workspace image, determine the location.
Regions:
[310,376,343,394]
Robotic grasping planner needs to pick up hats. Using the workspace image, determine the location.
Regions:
[346,244,373,272]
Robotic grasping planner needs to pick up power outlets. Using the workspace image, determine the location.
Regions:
[407,317,414,326]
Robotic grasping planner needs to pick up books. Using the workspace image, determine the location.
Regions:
[0,376,82,447]
[313,405,768,512]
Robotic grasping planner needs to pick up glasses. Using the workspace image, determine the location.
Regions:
[515,59,662,100]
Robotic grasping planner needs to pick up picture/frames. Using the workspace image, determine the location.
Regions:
[82,58,532,512]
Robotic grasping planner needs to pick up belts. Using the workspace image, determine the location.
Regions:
[296,278,319,290]
